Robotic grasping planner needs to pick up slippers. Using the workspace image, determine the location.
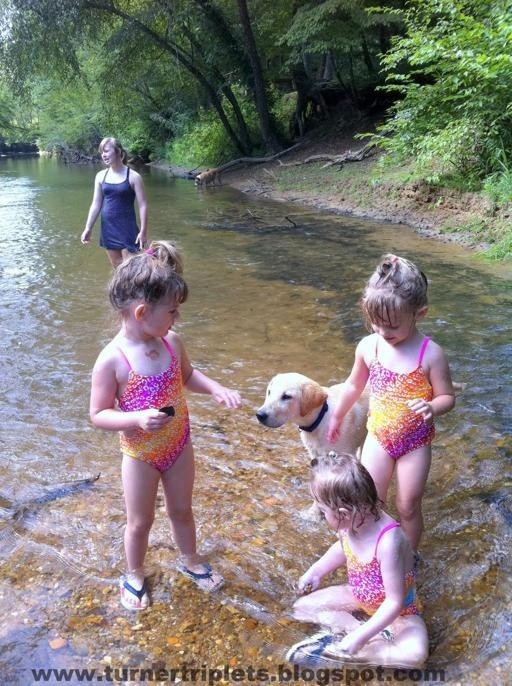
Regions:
[178,562,225,592]
[286,631,332,666]
[120,575,150,611]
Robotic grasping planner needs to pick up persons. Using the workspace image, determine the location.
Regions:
[326,253,456,568]
[81,137,148,269]
[89,239,241,611]
[286,451,431,668]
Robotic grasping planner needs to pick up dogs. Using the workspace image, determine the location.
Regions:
[255,372,372,461]
